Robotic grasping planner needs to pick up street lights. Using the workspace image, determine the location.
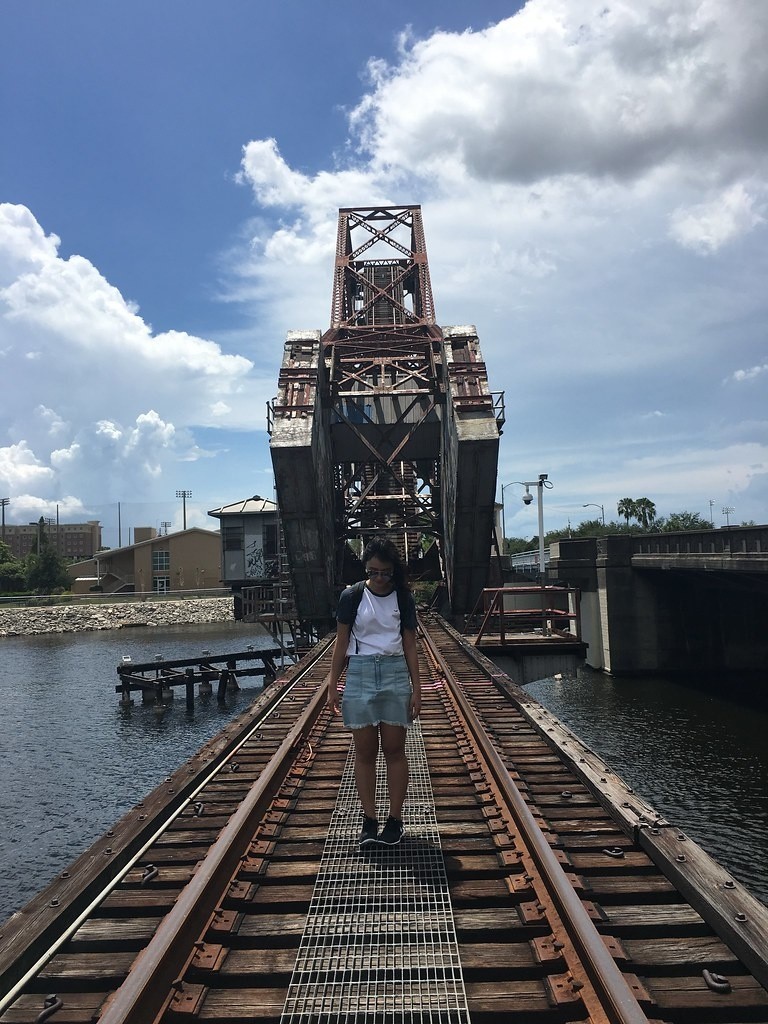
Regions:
[46,517,56,534]
[161,521,172,536]
[710,500,716,523]
[175,490,193,529]
[502,481,524,555]
[722,506,735,525]
[582,503,604,525]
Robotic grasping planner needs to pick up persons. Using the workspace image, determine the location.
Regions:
[328,537,423,847]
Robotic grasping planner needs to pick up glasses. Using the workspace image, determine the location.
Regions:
[365,568,392,578]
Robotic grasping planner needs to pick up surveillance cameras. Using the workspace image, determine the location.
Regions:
[522,494,533,505]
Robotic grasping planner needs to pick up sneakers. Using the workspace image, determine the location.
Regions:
[358,812,378,845]
[378,815,405,845]
